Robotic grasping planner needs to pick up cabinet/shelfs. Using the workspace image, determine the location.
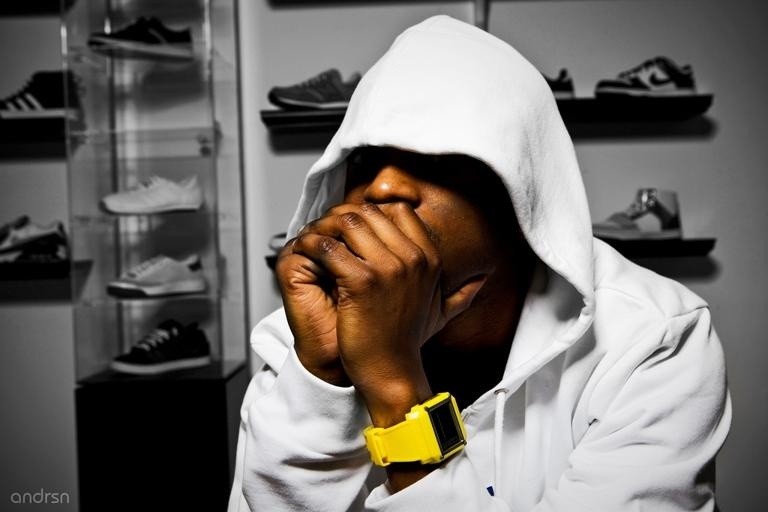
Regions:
[261,0,716,271]
[63,0,252,510]
[0,1,93,307]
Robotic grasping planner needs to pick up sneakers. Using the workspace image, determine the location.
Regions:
[0,215,69,281]
[106,254,206,299]
[595,55,694,94]
[101,175,204,214]
[543,69,573,98]
[111,318,211,376]
[269,70,360,109]
[0,70,79,119]
[89,15,193,58]
[592,188,682,240]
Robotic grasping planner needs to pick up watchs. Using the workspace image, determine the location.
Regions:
[364,392,465,469]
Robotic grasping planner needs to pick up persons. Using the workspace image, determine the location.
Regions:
[227,13,733,511]
